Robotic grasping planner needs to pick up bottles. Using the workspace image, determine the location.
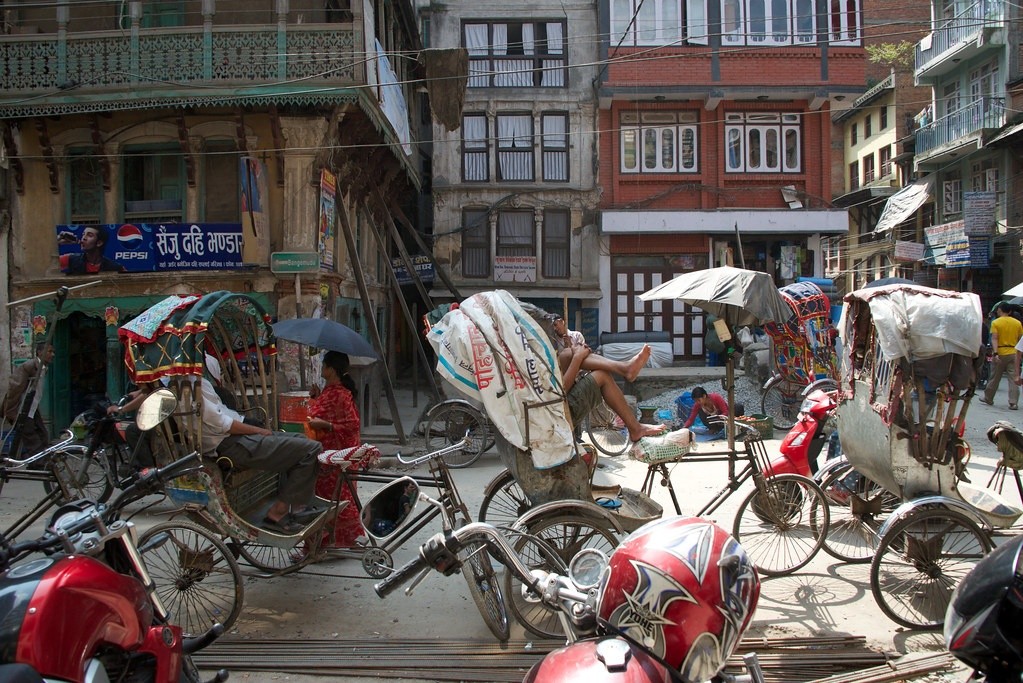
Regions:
[60,231,83,245]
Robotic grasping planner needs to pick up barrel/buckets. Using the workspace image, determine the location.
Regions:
[279,391,317,441]
[229,387,272,425]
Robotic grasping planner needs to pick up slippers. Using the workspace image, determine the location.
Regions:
[262,512,305,534]
[1008,403,1018,412]
[289,503,331,523]
[595,496,622,508]
[978,396,995,405]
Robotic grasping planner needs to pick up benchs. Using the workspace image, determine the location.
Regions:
[173,386,285,495]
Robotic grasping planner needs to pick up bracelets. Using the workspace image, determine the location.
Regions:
[329,423,333,432]
[118,406,123,413]
[994,353,998,356]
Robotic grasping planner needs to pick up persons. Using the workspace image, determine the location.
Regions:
[682,386,744,434]
[289,351,365,562]
[559,342,666,443]
[57,225,124,275]
[924,377,937,418]
[0,341,57,474]
[169,372,328,534]
[977,302,1023,410]
[550,313,587,438]
[106,381,180,471]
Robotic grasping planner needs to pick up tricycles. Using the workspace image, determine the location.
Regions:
[0,267,1023,683]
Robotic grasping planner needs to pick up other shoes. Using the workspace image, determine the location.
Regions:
[289,550,325,565]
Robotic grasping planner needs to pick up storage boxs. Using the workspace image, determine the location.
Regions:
[677,397,691,423]
[735,414,774,440]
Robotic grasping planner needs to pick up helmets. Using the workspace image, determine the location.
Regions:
[593,515,765,676]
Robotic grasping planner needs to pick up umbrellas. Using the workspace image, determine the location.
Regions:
[271,318,384,390]
